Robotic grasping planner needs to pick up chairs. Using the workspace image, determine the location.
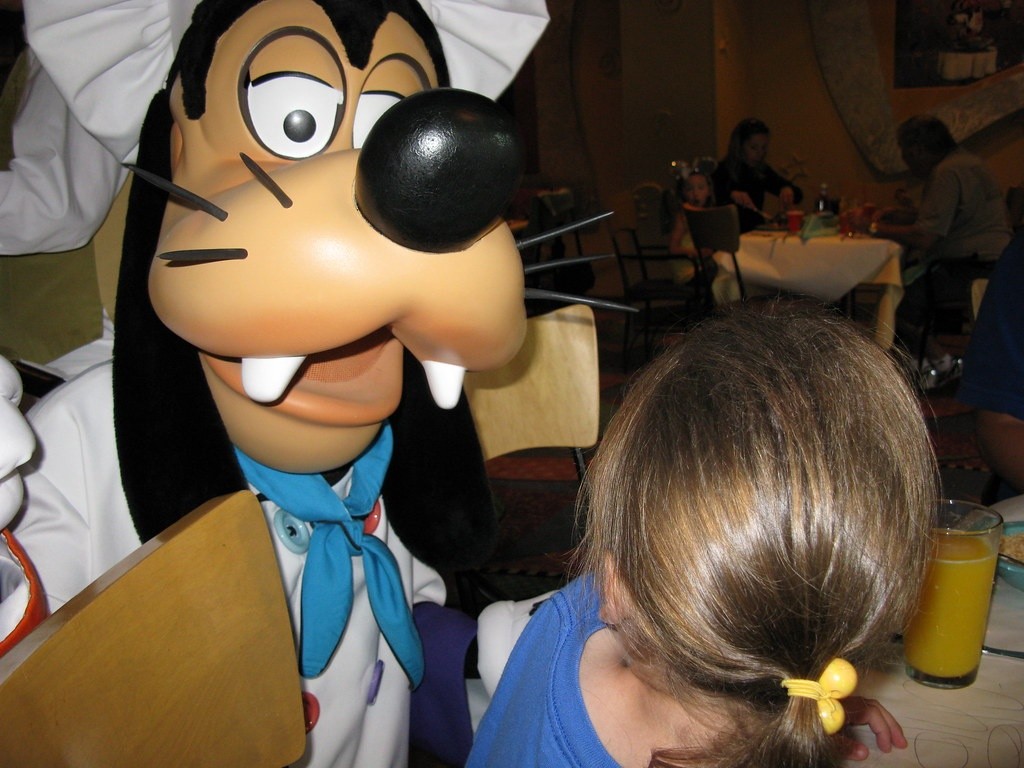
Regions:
[465,304,600,606]
[531,186,748,369]
[0,492,308,767]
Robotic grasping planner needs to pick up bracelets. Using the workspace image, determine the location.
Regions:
[868,223,877,234]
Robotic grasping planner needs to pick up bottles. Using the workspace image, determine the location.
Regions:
[815,182,829,213]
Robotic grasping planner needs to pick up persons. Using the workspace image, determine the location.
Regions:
[847,116,1014,375]
[670,157,715,287]
[467,289,942,767]
[959,230,1024,503]
[0,1,137,414]
[713,118,803,234]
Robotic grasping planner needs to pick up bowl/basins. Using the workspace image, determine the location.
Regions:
[997,521,1024,592]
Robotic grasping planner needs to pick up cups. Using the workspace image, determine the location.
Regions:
[901,499,1004,690]
[785,209,804,234]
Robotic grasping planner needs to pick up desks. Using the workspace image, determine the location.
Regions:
[852,488,1024,768]
[714,228,905,352]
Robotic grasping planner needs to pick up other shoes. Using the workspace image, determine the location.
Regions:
[919,352,963,390]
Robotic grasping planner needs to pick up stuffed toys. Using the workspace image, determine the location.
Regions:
[0,0,560,767]
